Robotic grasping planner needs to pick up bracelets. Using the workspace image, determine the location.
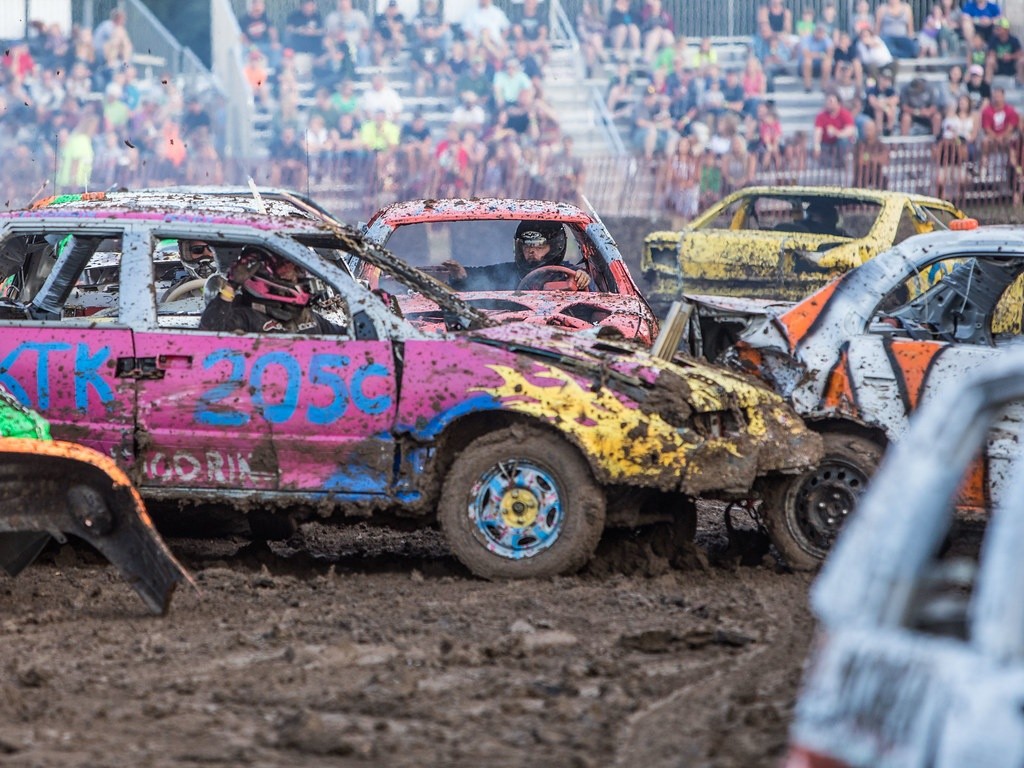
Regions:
[220,289,235,300]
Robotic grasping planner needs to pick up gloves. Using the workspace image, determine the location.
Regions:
[226,253,262,286]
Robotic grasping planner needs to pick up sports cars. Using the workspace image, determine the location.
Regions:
[3,187,825,581]
[645,184,1024,331]
[685,227,1024,573]
[784,346,1024,767]
[349,191,666,353]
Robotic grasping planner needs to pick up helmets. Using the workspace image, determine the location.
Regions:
[806,200,839,230]
[515,219,568,281]
[237,245,321,321]
[178,240,218,280]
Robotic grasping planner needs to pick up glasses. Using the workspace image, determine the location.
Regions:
[191,245,212,250]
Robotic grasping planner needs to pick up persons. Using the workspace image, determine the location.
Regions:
[439,218,598,293]
[0,235,348,337]
[0,0,1024,242]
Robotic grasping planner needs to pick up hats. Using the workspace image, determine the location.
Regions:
[993,17,1009,28]
[969,65,983,75]
